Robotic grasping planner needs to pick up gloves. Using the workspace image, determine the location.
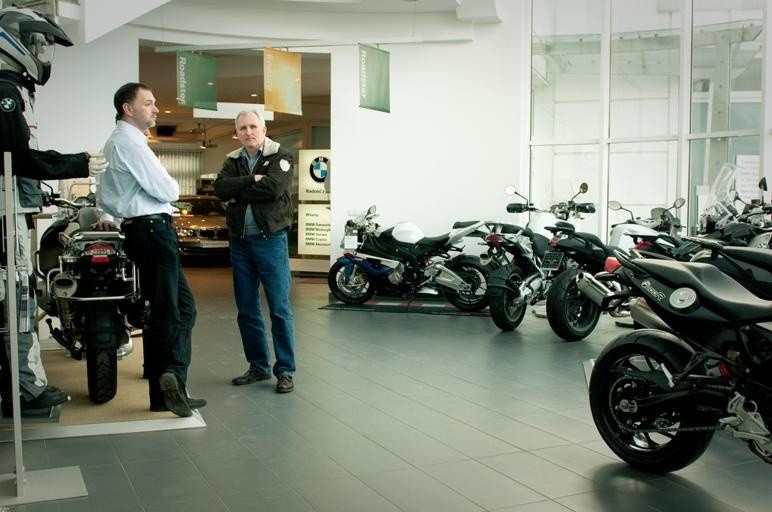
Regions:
[87,151,110,178]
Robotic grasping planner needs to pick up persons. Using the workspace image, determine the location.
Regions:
[95,81,209,420]
[0,4,109,415]
[214,105,298,394]
[91,113,150,379]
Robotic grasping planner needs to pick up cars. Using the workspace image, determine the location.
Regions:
[170,193,232,269]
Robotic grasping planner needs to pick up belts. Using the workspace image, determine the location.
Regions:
[126,212,167,224]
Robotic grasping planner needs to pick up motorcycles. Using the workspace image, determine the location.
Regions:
[586,248,772,476]
[477,163,772,340]
[30,179,151,405]
[327,203,494,314]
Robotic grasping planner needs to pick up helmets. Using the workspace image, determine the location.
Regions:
[0,4,74,85]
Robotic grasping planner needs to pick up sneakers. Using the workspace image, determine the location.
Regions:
[19,384,72,409]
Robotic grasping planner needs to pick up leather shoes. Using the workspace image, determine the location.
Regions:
[232,367,272,384]
[275,375,295,394]
[150,370,207,418]
